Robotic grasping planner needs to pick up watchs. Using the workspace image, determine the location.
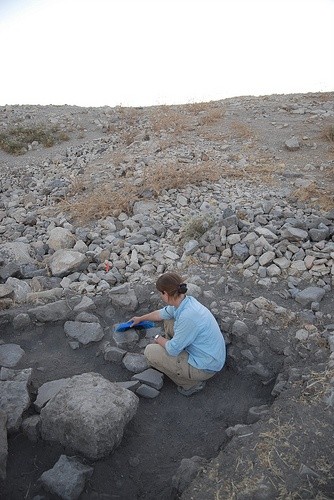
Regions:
[154,334,160,339]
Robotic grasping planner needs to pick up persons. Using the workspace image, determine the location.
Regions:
[126,272,227,397]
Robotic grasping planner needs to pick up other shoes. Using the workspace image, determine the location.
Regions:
[177,381,206,396]
